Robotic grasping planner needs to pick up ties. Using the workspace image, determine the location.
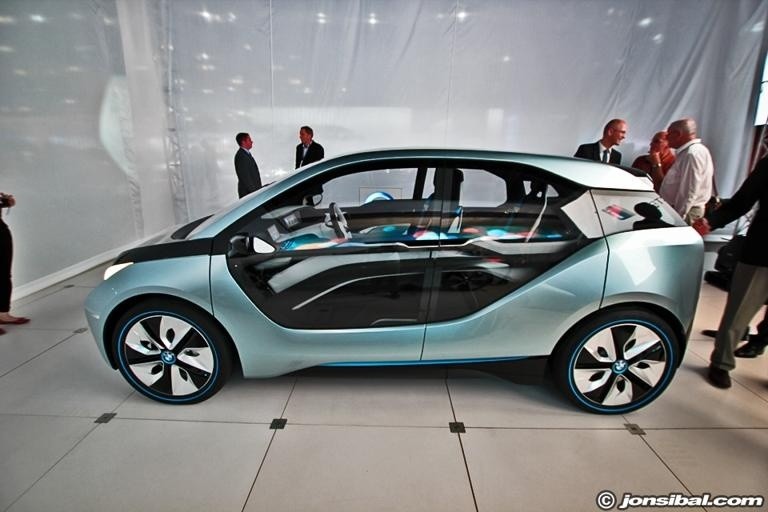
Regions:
[602,150,609,163]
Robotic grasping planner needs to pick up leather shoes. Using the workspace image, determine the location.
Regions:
[708,364,731,388]
[703,324,765,358]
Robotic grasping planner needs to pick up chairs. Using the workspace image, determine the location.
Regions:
[419,165,464,230]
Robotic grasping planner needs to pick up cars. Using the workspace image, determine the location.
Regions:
[81,149,706,415]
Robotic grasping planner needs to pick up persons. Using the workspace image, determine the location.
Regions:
[573,119,628,164]
[693,153,768,388]
[296,126,324,168]
[235,133,262,200]
[0,192,30,335]
[658,118,714,227]
[631,131,675,193]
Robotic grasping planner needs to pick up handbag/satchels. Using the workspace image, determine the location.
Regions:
[704,196,727,229]
[714,235,746,275]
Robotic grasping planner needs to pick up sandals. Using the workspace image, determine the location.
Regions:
[0,318,30,335]
